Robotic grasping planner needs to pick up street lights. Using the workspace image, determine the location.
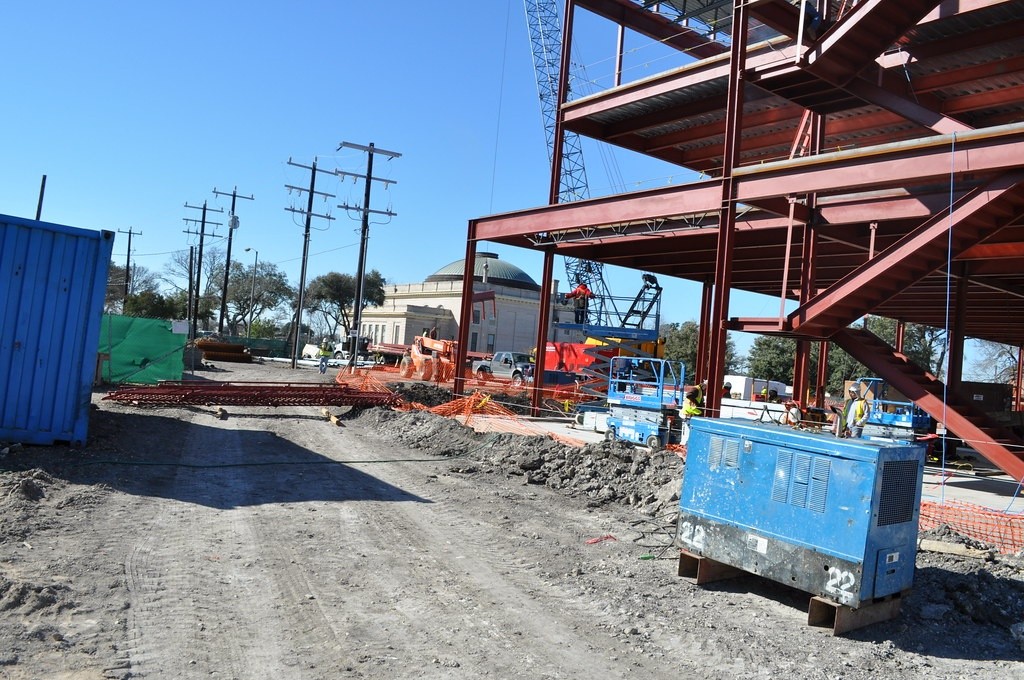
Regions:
[244,247,259,349]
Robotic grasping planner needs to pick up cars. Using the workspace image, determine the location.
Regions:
[303,343,350,364]
[199,331,214,337]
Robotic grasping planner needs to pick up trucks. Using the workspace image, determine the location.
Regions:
[723,373,786,400]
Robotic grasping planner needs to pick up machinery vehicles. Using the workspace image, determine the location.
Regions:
[399,334,470,382]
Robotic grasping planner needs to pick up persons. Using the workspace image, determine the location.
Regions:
[317,338,336,374]
[430,327,437,340]
[565,283,595,324]
[843,383,870,438]
[678,379,708,430]
[423,328,430,337]
[760,385,767,401]
[722,382,732,398]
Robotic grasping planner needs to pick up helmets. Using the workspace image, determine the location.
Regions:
[848,383,861,391]
[323,338,329,342]
[770,387,778,392]
[704,380,708,385]
[788,408,801,423]
[724,382,732,387]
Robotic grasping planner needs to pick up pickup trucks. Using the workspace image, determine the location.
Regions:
[472,350,536,390]
